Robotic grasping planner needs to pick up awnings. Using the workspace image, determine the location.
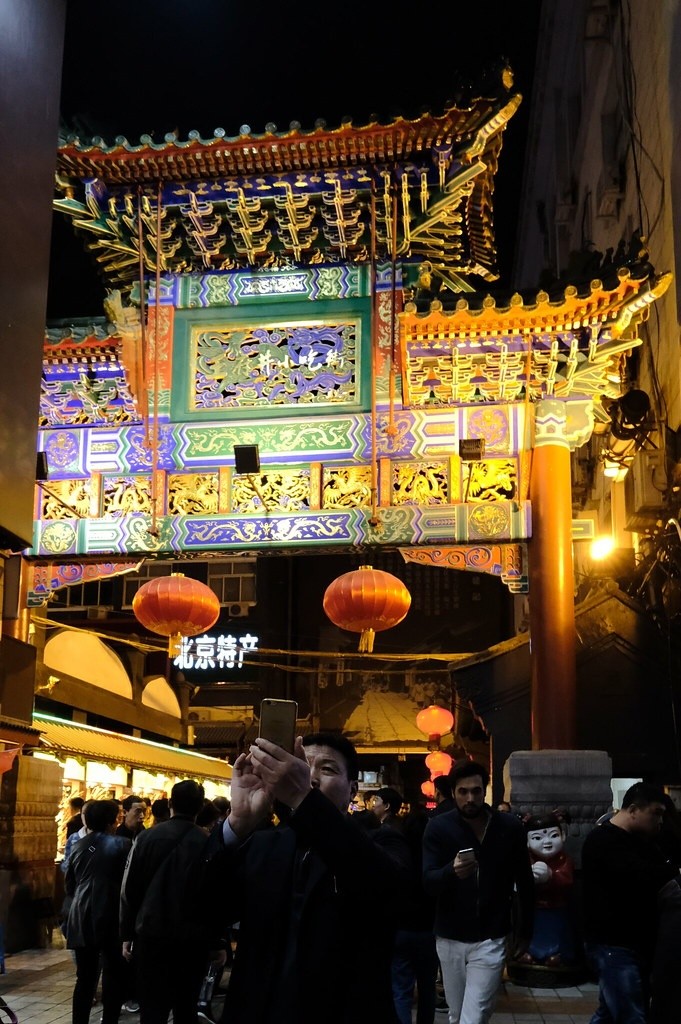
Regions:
[31,713,234,787]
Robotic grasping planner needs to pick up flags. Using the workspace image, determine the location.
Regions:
[0,748,20,774]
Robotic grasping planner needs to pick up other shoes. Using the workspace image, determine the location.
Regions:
[435,997,450,1012]
[196,1005,217,1024]
[121,1001,139,1012]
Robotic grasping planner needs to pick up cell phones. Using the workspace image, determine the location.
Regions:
[258,699,299,757]
[459,848,475,862]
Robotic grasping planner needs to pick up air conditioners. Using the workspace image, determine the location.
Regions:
[228,603,249,617]
[87,606,107,620]
[624,449,668,527]
[596,160,620,215]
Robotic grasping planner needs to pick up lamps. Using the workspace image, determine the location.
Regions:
[164,772,175,781]
[212,779,221,786]
[76,756,88,767]
[233,444,271,513]
[53,753,67,764]
[106,762,118,771]
[34,451,83,519]
[460,438,486,503]
[121,765,132,774]
[187,775,195,781]
[176,773,184,780]
[146,770,158,778]
[197,777,205,784]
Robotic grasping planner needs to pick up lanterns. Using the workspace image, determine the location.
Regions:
[324,566,411,653]
[133,573,221,658]
[416,705,455,809]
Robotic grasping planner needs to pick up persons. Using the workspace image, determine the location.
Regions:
[513,808,576,966]
[59,732,681,1024]
[410,676,451,710]
